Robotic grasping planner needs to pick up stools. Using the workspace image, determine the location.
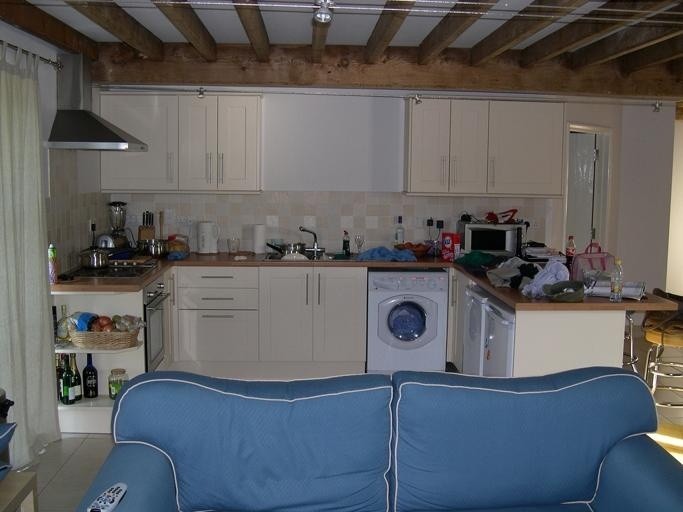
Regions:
[641,288,683,412]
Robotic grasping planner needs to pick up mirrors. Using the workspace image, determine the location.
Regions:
[565,124,618,256]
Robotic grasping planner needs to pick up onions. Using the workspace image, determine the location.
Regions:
[102,324,112,333]
[99,316,110,327]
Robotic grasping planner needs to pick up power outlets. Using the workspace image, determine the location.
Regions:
[593,148,599,160]
[592,228,599,239]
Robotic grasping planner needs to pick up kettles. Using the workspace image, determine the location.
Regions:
[76,246,114,269]
[196,221,220,254]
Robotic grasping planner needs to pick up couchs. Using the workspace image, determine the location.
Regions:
[77,367,683,512]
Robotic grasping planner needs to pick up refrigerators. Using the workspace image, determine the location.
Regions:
[482,296,516,378]
[462,284,493,376]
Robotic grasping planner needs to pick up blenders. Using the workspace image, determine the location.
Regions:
[105,201,131,248]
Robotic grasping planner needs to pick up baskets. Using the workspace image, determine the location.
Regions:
[68,327,139,351]
[395,243,431,257]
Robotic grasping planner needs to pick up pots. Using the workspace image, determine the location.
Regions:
[135,239,169,257]
[266,242,306,258]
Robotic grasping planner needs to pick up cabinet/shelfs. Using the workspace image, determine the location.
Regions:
[490,94,567,196]
[178,86,262,193]
[49,251,173,434]
[92,85,178,193]
[170,253,259,380]
[450,260,678,378]
[404,91,488,197]
[259,260,368,379]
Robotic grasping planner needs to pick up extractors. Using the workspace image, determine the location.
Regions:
[45,54,150,152]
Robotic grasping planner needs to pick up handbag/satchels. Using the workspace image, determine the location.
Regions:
[570,243,616,286]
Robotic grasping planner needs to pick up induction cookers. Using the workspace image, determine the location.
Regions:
[66,265,156,278]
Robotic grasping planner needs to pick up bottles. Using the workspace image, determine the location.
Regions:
[396,216,404,249]
[48,244,57,284]
[62,356,74,404]
[610,260,623,302]
[109,369,128,400]
[83,354,99,397]
[567,236,575,267]
[343,232,351,258]
[56,359,64,401]
[71,354,83,402]
[57,305,71,343]
[433,240,439,257]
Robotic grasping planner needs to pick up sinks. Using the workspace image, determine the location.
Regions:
[262,252,357,261]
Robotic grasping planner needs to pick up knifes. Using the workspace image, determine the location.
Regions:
[142,211,153,228]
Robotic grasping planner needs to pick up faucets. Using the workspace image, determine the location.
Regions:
[298,226,325,256]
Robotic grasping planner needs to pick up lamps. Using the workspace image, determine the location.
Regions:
[314,0,336,23]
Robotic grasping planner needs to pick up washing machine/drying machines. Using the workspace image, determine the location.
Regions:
[367,271,449,373]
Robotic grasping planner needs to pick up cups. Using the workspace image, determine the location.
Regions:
[354,232,363,256]
[226,238,240,254]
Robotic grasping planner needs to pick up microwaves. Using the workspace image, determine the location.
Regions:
[456,220,526,258]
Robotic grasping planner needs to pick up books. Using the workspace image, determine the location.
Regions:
[587,281,646,300]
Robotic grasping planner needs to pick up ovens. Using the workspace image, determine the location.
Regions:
[142,289,171,373]
